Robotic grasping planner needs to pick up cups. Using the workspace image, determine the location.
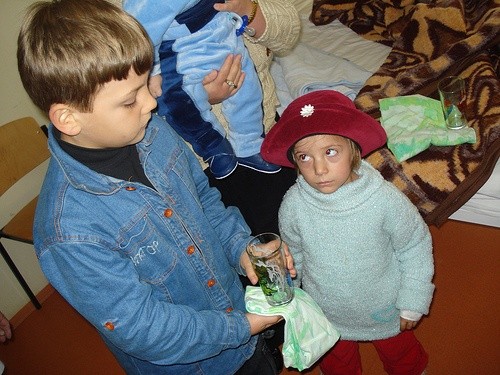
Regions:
[245,233,295,308]
[437,75,468,130]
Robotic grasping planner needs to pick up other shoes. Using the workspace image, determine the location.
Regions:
[236,152,282,173]
[203,138,238,179]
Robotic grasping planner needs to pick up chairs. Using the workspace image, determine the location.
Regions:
[1,117,53,312]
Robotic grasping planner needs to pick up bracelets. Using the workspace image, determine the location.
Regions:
[248,0,257,24]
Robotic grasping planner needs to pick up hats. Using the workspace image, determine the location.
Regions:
[260,89,387,168]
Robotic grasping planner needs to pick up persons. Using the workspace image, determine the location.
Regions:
[122,0,284,178]
[0,311,12,375]
[17,0,297,375]
[261,90,435,375]
[120,0,301,248]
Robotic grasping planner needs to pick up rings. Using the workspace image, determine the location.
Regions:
[225,80,232,86]
[232,84,237,88]
